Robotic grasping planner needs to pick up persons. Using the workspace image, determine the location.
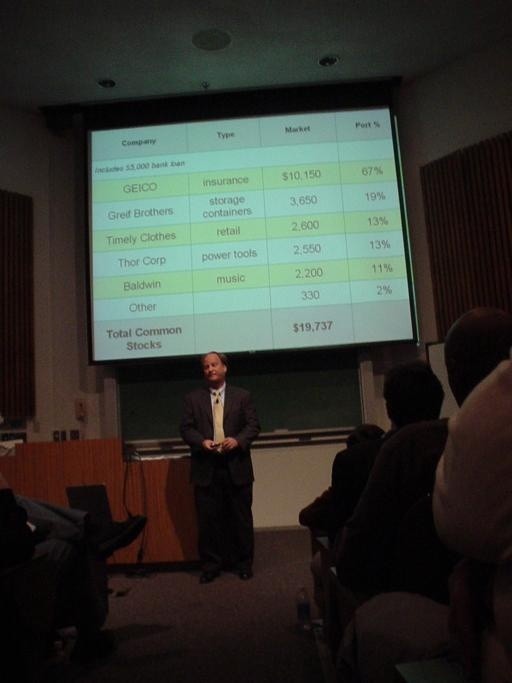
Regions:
[0,488,148,683]
[299,307,512,681]
[180,351,261,583]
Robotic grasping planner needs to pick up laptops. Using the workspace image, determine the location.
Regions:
[64,483,128,528]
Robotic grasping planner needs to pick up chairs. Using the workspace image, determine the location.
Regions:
[311,418,449,683]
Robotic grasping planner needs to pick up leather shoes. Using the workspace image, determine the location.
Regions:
[198,564,254,585]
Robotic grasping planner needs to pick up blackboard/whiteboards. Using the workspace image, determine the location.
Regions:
[118,345,363,451]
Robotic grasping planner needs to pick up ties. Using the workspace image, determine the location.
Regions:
[212,391,226,453]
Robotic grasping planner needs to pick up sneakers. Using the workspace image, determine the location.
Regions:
[88,513,148,559]
[70,628,117,664]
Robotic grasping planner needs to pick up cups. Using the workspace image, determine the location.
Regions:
[296,584,311,633]
